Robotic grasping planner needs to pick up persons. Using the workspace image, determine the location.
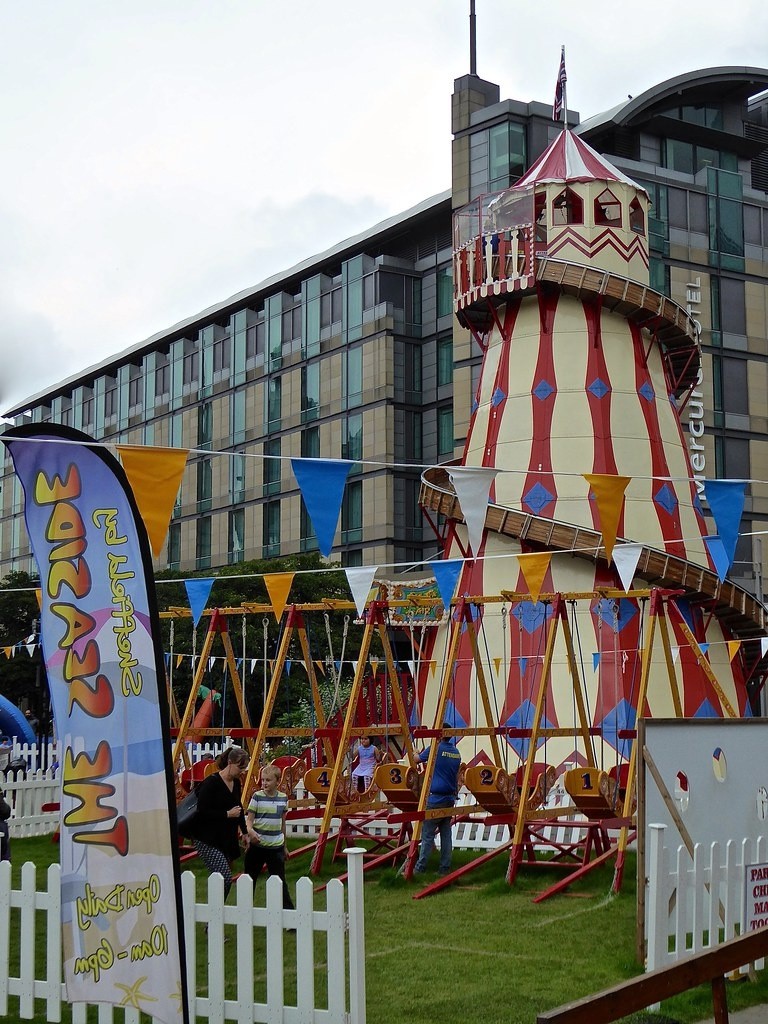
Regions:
[193,747,251,944]
[414,722,462,875]
[349,734,381,801]
[237,766,298,932]
[0,703,43,783]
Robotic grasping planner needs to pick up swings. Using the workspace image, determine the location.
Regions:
[167,596,647,818]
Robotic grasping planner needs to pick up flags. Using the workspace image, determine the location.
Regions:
[552,50,567,122]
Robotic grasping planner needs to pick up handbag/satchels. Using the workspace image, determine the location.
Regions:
[176,773,221,839]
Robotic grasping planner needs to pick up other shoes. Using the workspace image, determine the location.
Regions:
[434,869,449,876]
[401,867,420,875]
